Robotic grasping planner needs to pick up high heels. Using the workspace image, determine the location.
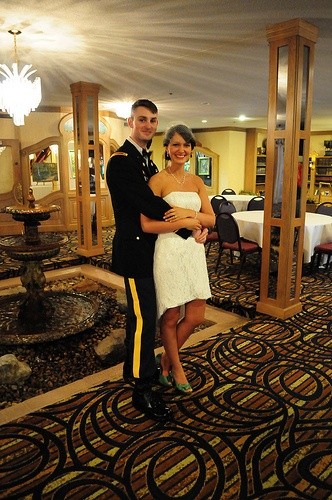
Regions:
[169,371,193,394]
[155,353,172,387]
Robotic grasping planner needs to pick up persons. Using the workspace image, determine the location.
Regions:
[140,125,215,395]
[106,99,203,420]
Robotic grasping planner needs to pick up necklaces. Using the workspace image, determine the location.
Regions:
[166,165,186,184]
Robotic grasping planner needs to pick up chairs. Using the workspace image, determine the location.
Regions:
[209,188,332,282]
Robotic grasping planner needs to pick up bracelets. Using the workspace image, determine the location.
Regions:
[193,210,197,219]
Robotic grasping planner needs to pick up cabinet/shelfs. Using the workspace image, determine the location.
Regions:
[255,154,332,189]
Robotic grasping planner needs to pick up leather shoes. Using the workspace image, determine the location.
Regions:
[133,388,171,422]
[125,376,135,387]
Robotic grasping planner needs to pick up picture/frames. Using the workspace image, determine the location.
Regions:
[197,157,209,175]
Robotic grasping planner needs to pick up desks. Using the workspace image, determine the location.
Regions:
[208,195,265,213]
[228,210,332,277]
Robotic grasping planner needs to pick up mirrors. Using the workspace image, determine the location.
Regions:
[162,146,220,195]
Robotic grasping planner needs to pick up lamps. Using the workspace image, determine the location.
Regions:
[0,28,42,126]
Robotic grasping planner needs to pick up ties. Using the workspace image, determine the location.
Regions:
[142,149,148,159]
